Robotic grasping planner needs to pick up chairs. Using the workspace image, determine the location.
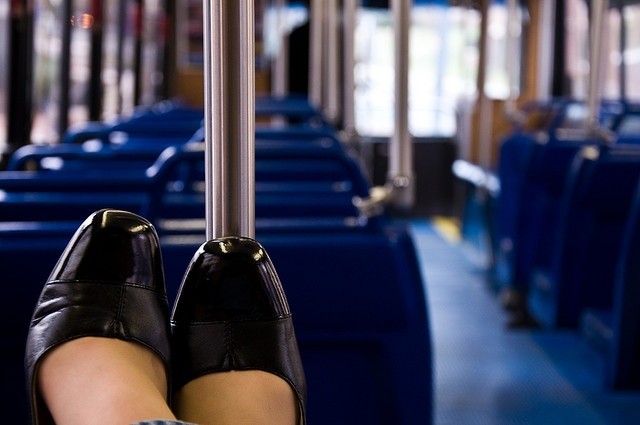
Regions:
[0,94,434,425]
[454,98,640,388]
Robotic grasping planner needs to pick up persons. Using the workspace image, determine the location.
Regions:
[22,208,311,425]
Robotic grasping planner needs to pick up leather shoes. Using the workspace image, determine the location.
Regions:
[23,209,173,424]
[169,236,307,425]
